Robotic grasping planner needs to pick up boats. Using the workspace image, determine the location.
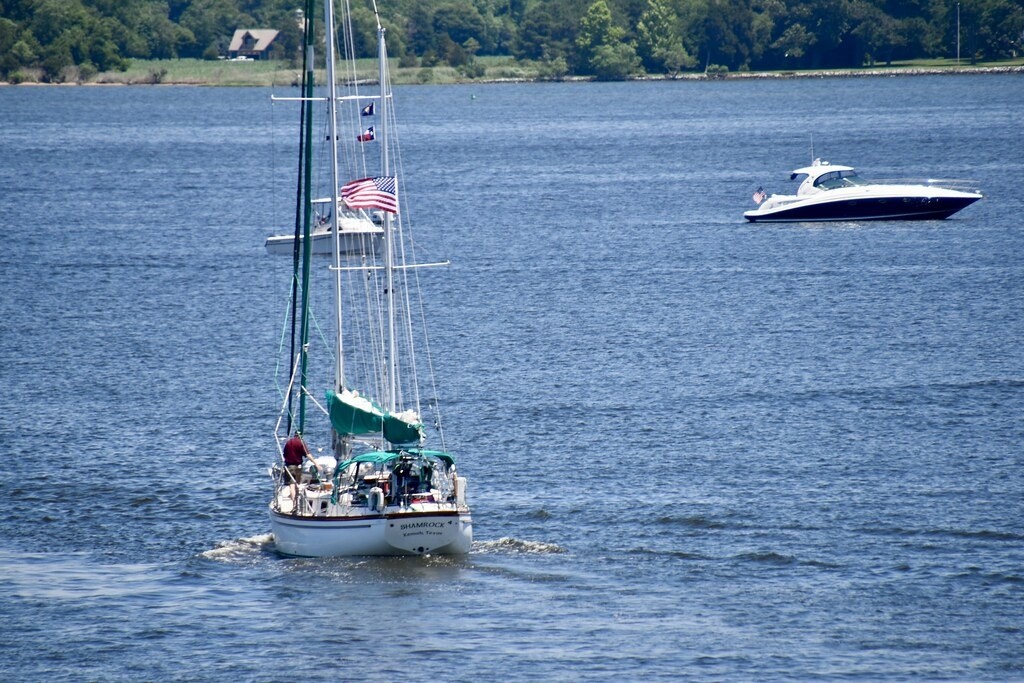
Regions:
[742,132,985,225]
[265,195,388,255]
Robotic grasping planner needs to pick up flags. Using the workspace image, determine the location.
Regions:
[341,176,398,213]
[360,102,373,116]
[752,187,765,204]
[357,126,374,142]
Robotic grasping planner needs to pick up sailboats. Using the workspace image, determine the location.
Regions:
[265,1,473,559]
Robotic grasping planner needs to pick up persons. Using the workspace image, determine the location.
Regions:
[393,458,432,506]
[283,430,323,513]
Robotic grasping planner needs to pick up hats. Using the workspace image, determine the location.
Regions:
[295,430,302,436]
